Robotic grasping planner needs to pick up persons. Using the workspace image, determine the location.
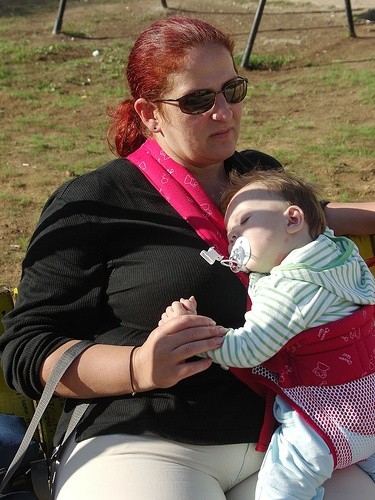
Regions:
[0,15,375,499]
[152,166,374,500]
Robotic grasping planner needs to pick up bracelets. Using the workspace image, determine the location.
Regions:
[129,347,137,397]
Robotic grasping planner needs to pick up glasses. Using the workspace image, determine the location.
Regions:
[148,75,250,116]
